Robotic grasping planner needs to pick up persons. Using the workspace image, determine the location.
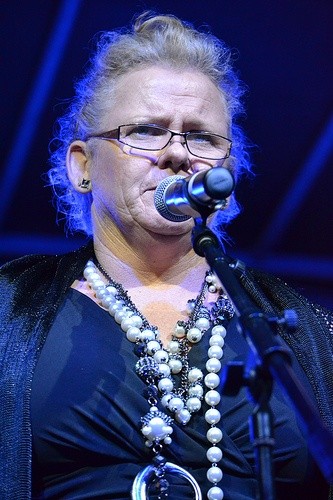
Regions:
[1,14,333,499]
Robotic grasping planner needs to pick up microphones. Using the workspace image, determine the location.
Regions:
[153,167,235,222]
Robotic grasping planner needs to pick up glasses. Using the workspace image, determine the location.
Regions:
[84,124,232,160]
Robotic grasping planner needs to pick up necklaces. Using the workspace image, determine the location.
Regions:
[84,261,234,499]
